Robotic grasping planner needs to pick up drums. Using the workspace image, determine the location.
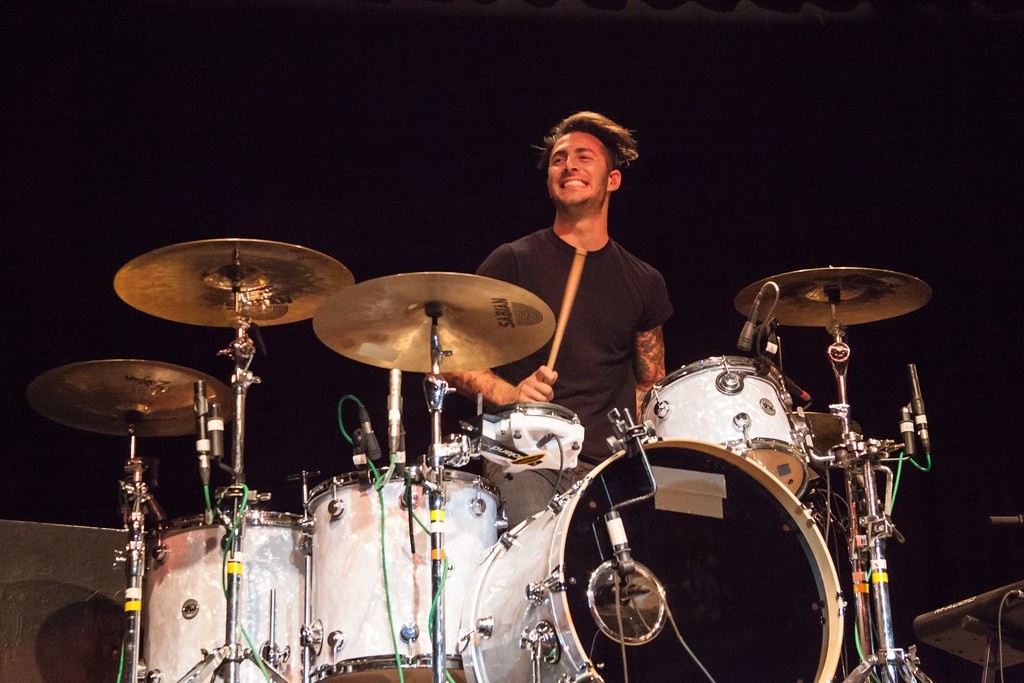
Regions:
[301,464,511,683]
[455,436,849,682]
[123,508,315,683]
[635,350,813,501]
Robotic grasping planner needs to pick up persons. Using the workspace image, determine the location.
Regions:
[431,112,672,541]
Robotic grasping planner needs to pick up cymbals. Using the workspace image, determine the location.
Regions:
[780,405,868,454]
[113,235,357,328]
[733,260,935,331]
[312,271,559,376]
[22,357,238,441]
